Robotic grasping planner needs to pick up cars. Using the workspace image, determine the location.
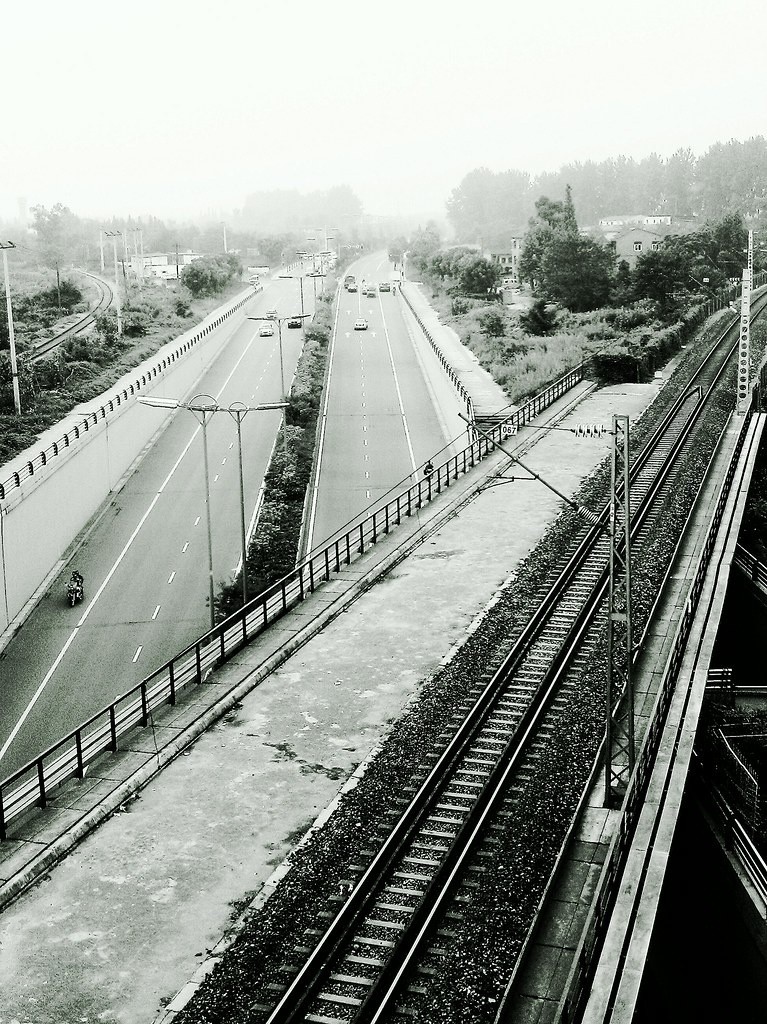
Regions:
[344,275,391,298]
[287,313,302,329]
[259,324,276,337]
[354,317,369,331]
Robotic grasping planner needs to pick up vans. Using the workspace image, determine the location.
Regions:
[503,279,517,290]
[249,275,260,286]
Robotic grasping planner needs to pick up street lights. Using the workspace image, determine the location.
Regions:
[306,225,339,259]
[278,273,327,341]
[247,313,311,452]
[137,393,290,629]
[296,251,331,306]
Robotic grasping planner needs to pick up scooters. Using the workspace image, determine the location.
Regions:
[424,461,434,482]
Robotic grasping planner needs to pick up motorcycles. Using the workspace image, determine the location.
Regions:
[64,580,83,607]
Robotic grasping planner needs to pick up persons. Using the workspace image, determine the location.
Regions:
[424,460,434,475]
[65,569,84,600]
